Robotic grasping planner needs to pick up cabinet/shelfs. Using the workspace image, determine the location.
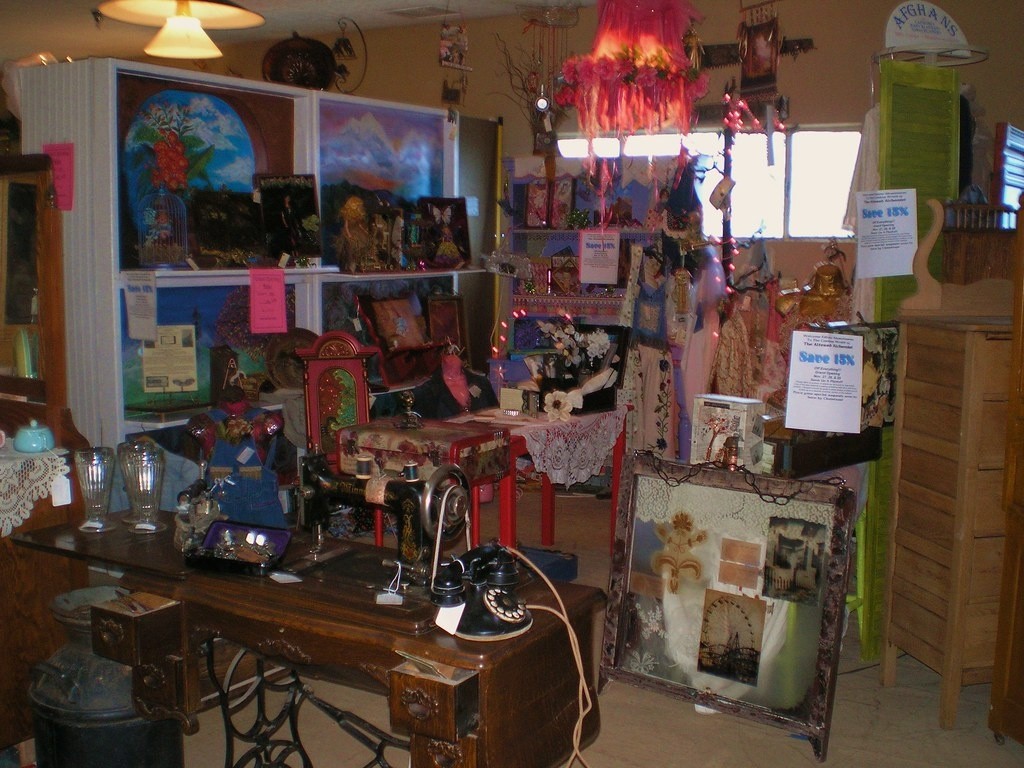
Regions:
[22,56,465,454]
[882,310,1024,730]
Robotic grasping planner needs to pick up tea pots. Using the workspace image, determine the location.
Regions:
[13,420,55,452]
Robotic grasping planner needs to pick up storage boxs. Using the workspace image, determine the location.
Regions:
[335,416,513,485]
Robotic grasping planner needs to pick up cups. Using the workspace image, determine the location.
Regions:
[117,441,156,523]
[124,447,167,533]
[75,447,118,532]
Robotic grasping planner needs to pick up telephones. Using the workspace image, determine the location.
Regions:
[432,542,535,642]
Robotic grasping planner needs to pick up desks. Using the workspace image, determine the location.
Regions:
[332,405,635,554]
[12,505,607,768]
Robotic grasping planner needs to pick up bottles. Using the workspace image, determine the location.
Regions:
[31,288,38,323]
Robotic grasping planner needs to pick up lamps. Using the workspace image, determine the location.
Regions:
[553,0,707,253]
[98,0,266,59]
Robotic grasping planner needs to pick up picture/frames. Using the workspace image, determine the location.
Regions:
[591,446,852,766]
[294,330,378,464]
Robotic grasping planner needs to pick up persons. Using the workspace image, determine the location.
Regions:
[333,195,380,275]
[411,352,501,507]
[430,224,470,271]
